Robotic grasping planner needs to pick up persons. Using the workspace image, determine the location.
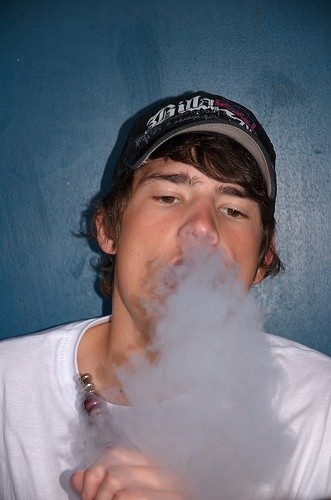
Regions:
[0,90,329,500]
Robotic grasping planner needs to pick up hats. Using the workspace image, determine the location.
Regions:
[111,88,277,199]
